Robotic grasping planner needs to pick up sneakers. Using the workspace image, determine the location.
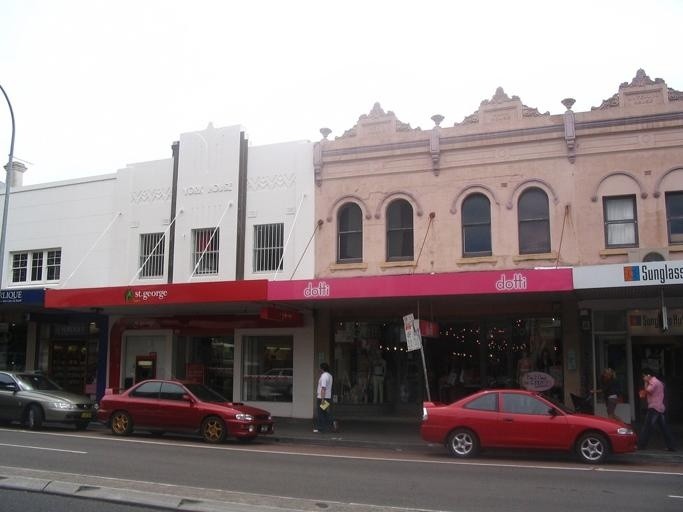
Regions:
[633,443,678,452]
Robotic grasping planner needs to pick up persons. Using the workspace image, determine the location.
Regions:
[369,352,387,405]
[637,368,676,450]
[314,362,338,432]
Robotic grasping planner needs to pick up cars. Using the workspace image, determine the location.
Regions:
[419,388,639,465]
[263,368,293,393]
[0,370,275,444]
